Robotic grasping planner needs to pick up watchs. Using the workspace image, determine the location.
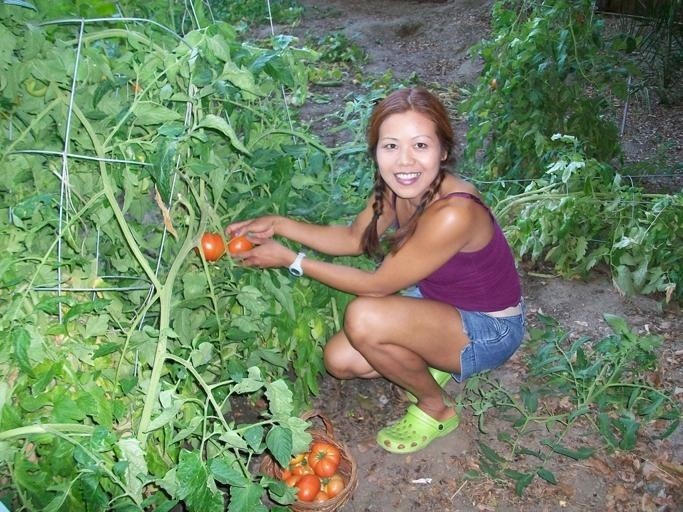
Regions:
[288,252,306,277]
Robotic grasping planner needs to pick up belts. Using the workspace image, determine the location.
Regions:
[484,304,521,318]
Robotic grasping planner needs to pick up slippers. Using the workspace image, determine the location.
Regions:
[405,366,453,404]
[376,403,460,454]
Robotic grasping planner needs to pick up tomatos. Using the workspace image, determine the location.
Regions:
[228,237,252,254]
[280,442,344,505]
[193,232,225,260]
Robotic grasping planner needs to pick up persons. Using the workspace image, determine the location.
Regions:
[224,87,525,454]
